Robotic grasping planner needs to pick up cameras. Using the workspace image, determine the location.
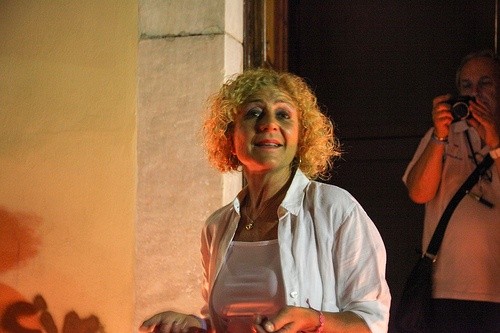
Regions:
[439,95,476,123]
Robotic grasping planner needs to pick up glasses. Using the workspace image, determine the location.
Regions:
[469,152,492,182]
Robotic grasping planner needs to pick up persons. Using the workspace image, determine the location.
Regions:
[403,49,500,333]
[139,68,391,333]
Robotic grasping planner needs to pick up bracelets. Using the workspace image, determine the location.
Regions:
[306,297,326,333]
[431,132,449,145]
[191,314,207,333]
[489,148,500,160]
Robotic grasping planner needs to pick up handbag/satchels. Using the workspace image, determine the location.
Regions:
[396,256,433,333]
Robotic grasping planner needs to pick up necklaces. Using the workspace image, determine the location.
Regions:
[245,187,287,231]
